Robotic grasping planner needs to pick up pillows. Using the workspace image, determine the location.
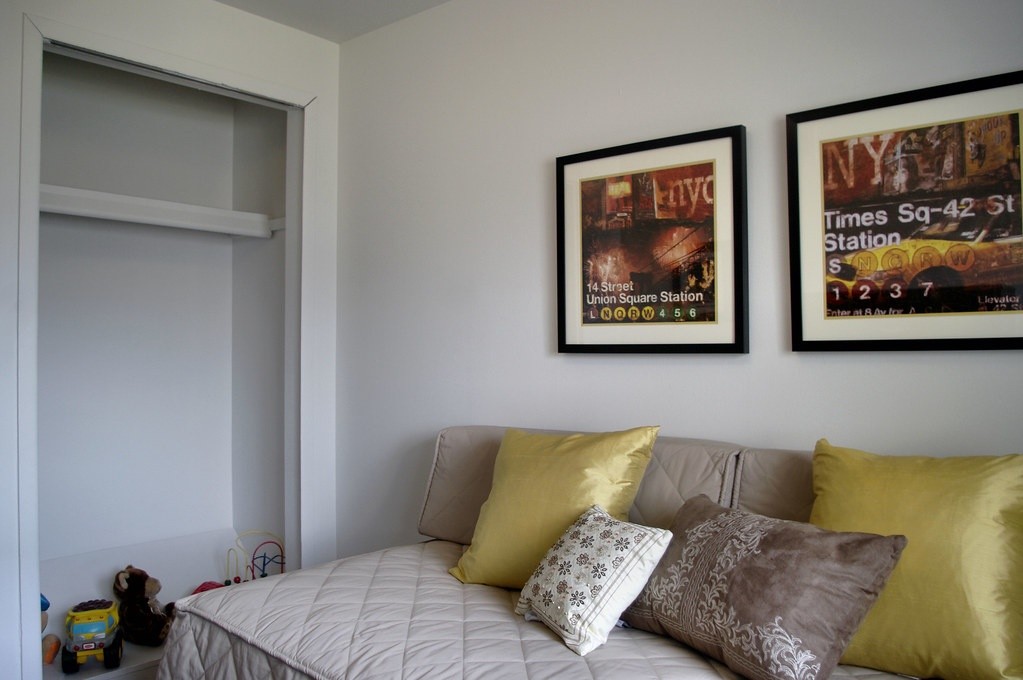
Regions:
[447,426,661,589]
[514,504,674,657]
[621,494,909,680]
[812,438,1023,680]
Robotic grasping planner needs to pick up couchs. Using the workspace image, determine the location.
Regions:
[158,427,945,680]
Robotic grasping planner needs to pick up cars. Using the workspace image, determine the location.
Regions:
[61,599,125,676]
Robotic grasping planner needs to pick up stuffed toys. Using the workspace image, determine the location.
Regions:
[112,565,177,648]
[39,592,62,666]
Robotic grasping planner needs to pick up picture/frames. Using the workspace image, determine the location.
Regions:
[786,71,1023,350]
[556,125,750,353]
